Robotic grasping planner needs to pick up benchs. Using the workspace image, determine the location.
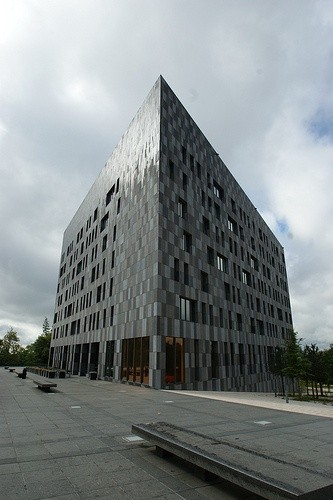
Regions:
[33,379,57,392]
[132,420,332,500]
